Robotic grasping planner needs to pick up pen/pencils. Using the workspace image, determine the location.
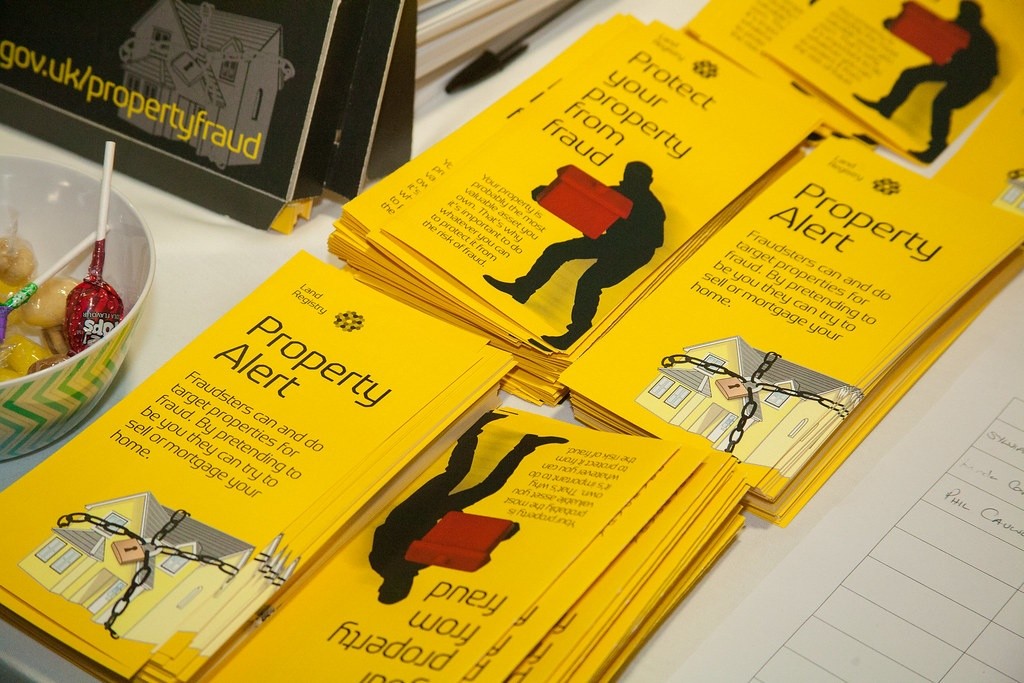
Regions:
[445,0,616,95]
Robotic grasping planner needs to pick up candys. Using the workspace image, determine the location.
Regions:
[0,235,123,382]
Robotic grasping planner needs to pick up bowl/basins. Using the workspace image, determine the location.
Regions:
[0,155,156,460]
